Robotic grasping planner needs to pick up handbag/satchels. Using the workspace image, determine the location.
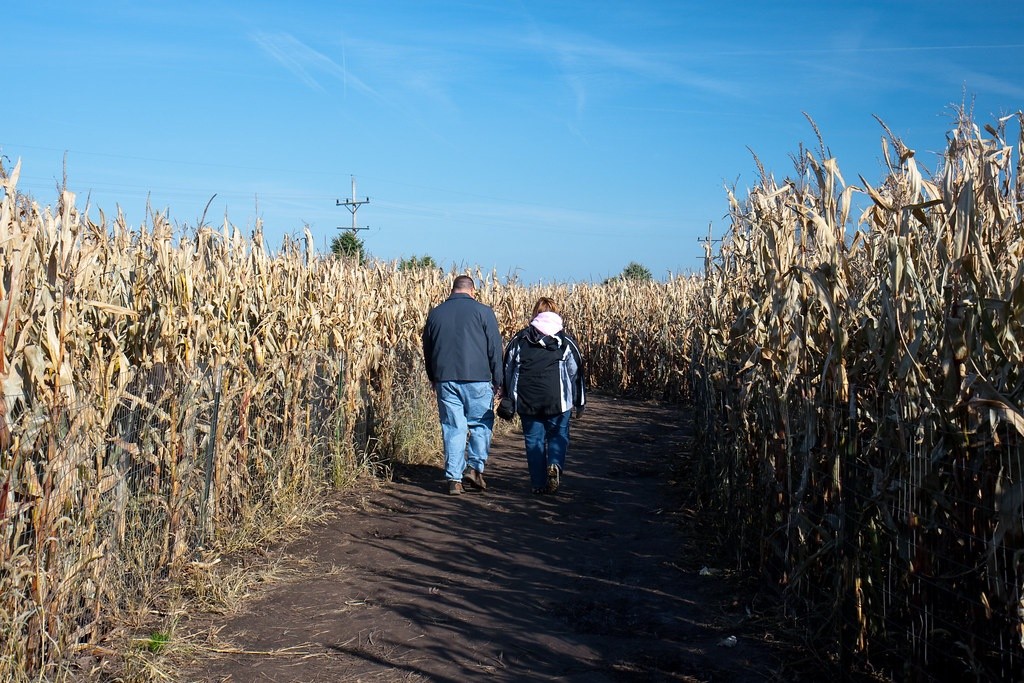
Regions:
[497,395,516,420]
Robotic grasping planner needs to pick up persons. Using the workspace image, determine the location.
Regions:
[422,276,504,495]
[502,297,586,495]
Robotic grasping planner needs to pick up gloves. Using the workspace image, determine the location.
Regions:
[576,406,584,418]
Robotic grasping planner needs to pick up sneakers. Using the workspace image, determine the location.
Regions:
[448,481,465,494]
[532,488,543,494]
[547,464,559,493]
[464,467,486,490]
